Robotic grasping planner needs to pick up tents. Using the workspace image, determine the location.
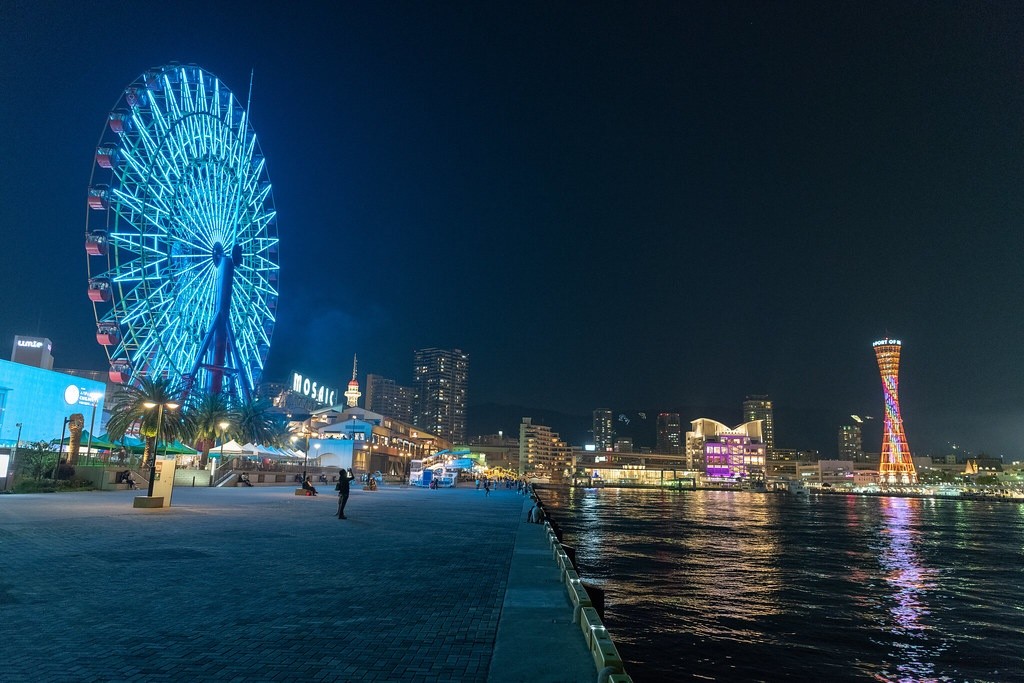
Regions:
[207,438,246,463]
[52,430,116,467]
[241,442,311,466]
[97,432,197,468]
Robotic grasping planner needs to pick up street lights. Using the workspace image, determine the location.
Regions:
[133,400,180,508]
[86,392,103,465]
[362,443,379,490]
[219,422,230,465]
[399,449,412,488]
[295,425,319,496]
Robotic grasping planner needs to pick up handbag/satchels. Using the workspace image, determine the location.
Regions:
[335,483,341,491]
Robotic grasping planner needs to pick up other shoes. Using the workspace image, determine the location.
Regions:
[316,492,318,493]
[343,513,344,514]
[337,513,347,519]
[313,495,316,496]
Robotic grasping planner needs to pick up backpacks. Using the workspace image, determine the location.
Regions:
[302,482,308,489]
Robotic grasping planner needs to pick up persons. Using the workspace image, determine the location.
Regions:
[305,477,318,496]
[241,473,254,486]
[119,446,127,467]
[474,478,527,496]
[295,474,304,483]
[430,478,438,489]
[531,502,544,523]
[320,474,328,485]
[337,469,354,520]
